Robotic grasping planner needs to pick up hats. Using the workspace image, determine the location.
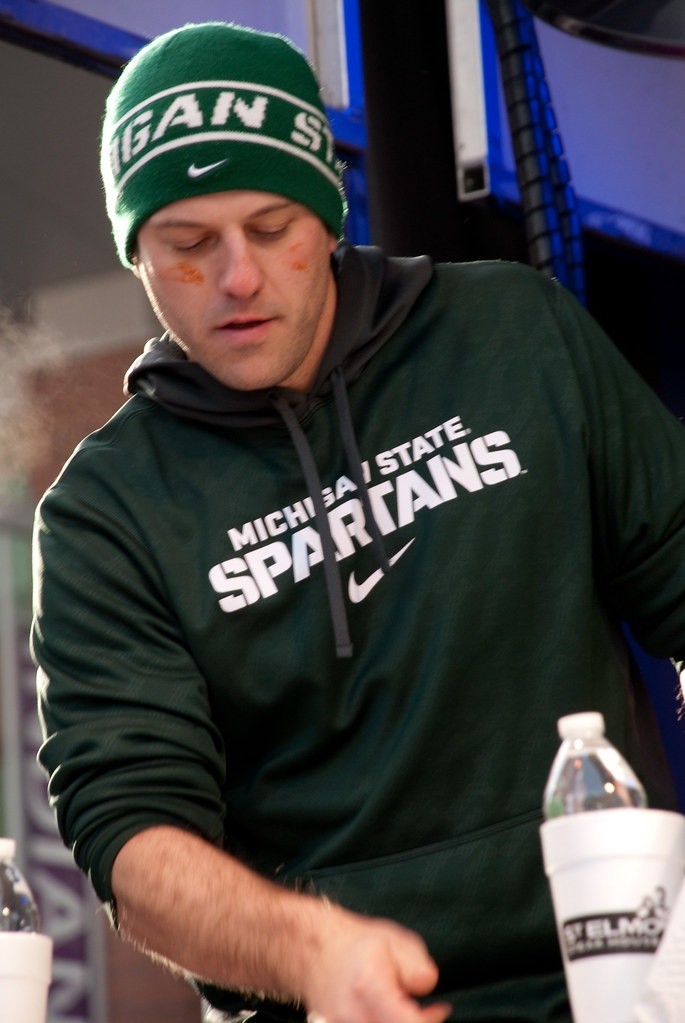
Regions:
[100,22,346,269]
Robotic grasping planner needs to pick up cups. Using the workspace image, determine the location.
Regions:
[538,807,685,1022]
[1,837,42,934]
[0,928,54,1023]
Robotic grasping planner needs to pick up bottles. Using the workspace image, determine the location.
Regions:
[538,710,648,825]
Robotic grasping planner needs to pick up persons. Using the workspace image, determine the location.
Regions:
[33,23,685,1023]
